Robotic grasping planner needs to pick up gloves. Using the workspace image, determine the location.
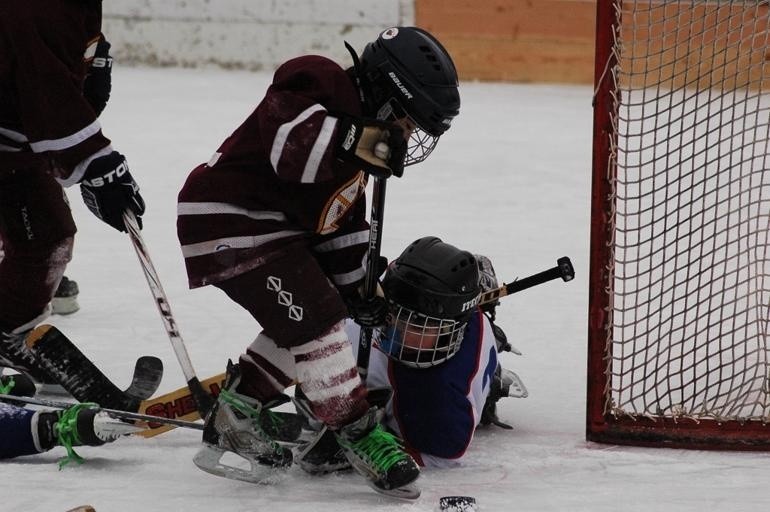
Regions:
[333,262,385,328]
[335,117,406,180]
[78,151,145,232]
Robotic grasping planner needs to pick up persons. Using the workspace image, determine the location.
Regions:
[1,1,529,489]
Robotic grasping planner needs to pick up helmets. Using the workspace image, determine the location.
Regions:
[371,237,481,368]
[356,27,462,165]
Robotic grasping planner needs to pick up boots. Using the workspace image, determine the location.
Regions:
[1,334,61,386]
[201,360,302,468]
[28,403,104,455]
[336,407,421,491]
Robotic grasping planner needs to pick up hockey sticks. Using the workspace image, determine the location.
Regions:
[24,256,575,437]
[121,207,313,442]
